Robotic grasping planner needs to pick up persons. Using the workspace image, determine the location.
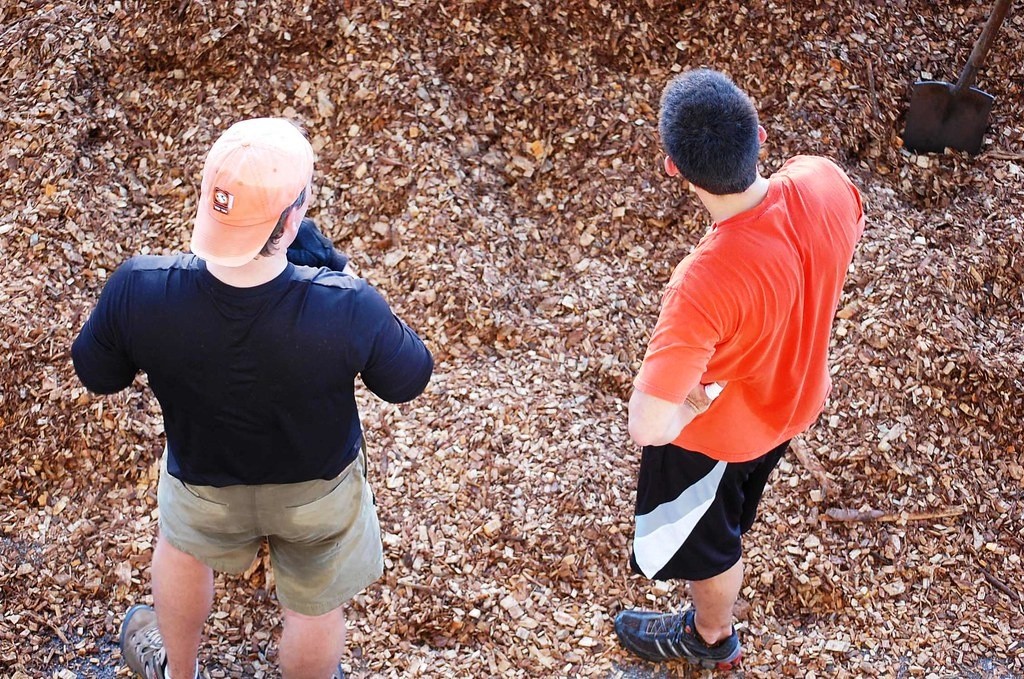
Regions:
[72,116,435,679]
[613,68,866,670]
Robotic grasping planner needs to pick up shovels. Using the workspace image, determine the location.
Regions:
[903,0,1011,157]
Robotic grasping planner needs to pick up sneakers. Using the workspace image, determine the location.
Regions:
[329,661,346,679]
[614,610,741,670]
[120,604,201,678]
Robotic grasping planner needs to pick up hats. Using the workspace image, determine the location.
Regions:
[189,117,314,266]
